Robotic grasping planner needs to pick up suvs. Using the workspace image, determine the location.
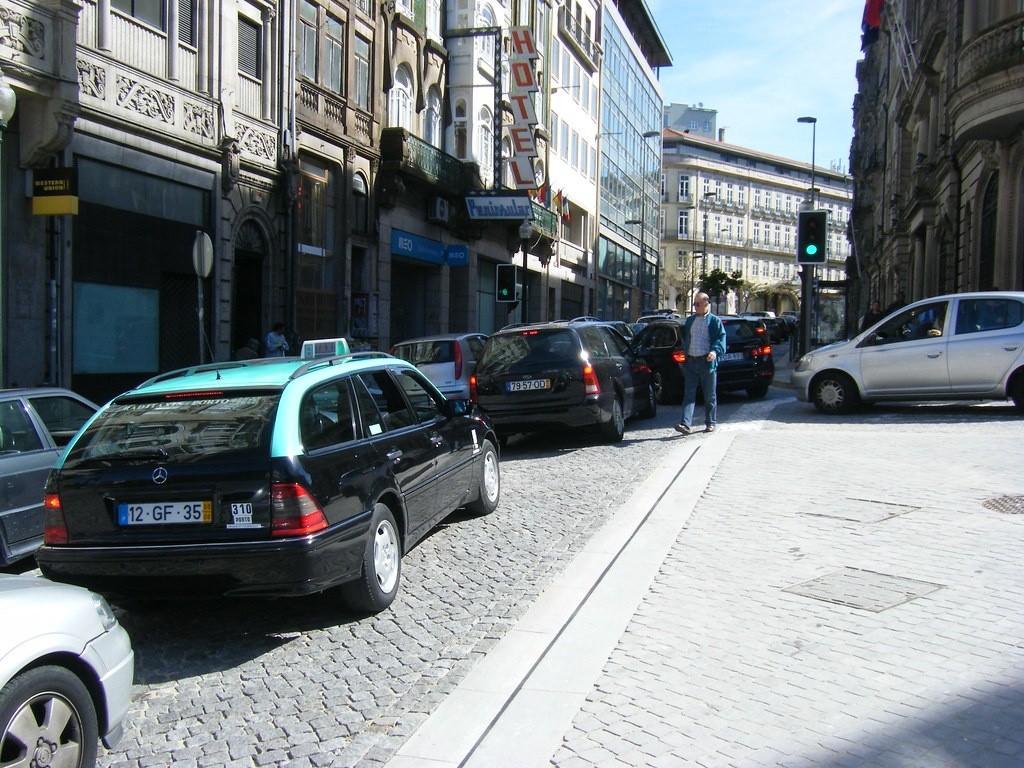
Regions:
[635,308,684,326]
[469,314,656,456]
[629,314,775,406]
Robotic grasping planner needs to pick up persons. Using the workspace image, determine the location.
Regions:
[860,291,910,341]
[675,293,727,435]
[235,339,260,360]
[264,322,289,358]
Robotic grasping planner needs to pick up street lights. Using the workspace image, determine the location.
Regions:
[796,115,818,355]
[624,131,661,314]
[519,219,533,324]
[0,69,16,390]
[686,191,718,280]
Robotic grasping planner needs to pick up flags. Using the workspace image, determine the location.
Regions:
[553,190,563,216]
[537,183,546,204]
[563,197,570,222]
[543,186,551,208]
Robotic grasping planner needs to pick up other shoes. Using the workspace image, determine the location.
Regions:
[675,423,692,435]
[705,425,716,432]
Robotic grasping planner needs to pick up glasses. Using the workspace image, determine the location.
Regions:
[693,301,706,307]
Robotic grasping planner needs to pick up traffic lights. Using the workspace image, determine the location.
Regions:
[797,211,828,265]
[496,264,516,302]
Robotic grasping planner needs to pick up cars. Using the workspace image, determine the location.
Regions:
[0,386,101,573]
[386,332,489,402]
[34,339,500,621]
[593,319,648,344]
[0,571,134,768]
[791,291,1024,416]
[721,309,804,345]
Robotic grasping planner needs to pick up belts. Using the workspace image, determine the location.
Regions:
[687,354,708,359]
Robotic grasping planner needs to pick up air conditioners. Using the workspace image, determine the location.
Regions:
[428,199,449,222]
